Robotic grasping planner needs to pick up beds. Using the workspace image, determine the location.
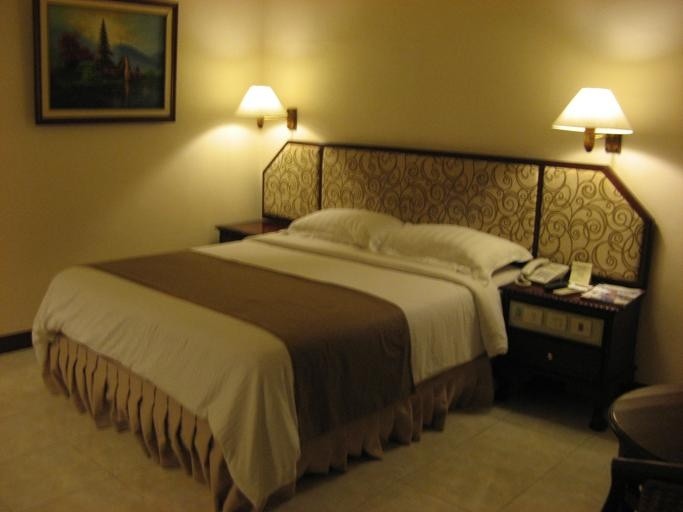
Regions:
[29,138,660,511]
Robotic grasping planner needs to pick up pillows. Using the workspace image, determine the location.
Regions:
[286,206,403,254]
[379,222,534,287]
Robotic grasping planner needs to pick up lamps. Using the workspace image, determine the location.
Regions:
[233,84,299,132]
[549,86,636,155]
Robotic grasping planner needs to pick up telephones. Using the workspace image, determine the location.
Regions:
[514,257,569,287]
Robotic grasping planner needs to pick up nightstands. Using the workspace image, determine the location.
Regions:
[214,218,288,245]
[492,279,647,432]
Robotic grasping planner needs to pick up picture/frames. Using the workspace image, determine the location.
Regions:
[30,1,182,128]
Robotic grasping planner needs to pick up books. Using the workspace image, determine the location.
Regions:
[580,283,644,308]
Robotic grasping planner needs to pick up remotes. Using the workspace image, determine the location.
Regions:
[544,282,567,292]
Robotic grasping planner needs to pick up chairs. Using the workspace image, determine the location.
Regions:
[608,453,683,512]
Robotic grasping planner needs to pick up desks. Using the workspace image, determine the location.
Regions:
[606,381,683,464]
